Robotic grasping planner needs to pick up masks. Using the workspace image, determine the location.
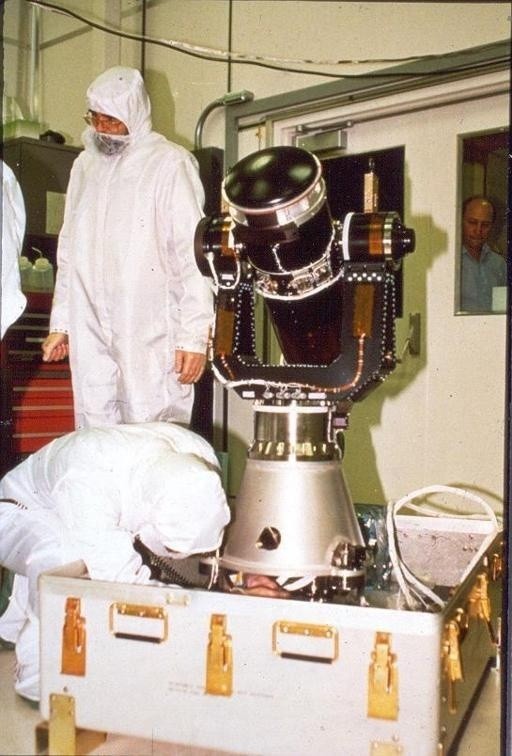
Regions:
[89,131,130,153]
[140,525,191,561]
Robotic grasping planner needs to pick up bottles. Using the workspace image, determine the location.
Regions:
[31,258,54,292]
[18,255,32,293]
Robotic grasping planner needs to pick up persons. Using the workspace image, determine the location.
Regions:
[460,195,508,312]
[38,66,221,432]
[3,159,30,345]
[0,420,291,713]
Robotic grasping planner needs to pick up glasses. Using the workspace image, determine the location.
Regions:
[84,112,121,128]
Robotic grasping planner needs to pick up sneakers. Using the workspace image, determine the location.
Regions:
[15,666,38,711]
[0,617,30,652]
